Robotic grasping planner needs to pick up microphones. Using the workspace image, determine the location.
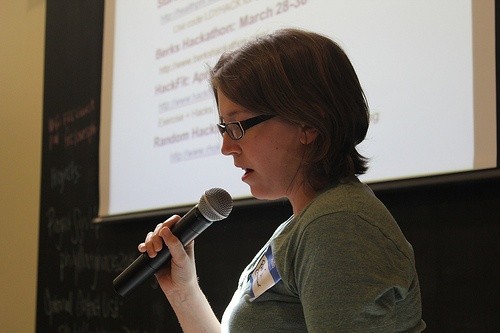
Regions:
[109,188,234,296]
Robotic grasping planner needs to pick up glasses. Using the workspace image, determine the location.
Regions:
[216,113,279,140]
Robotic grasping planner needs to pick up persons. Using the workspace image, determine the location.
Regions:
[133,27,427,333]
